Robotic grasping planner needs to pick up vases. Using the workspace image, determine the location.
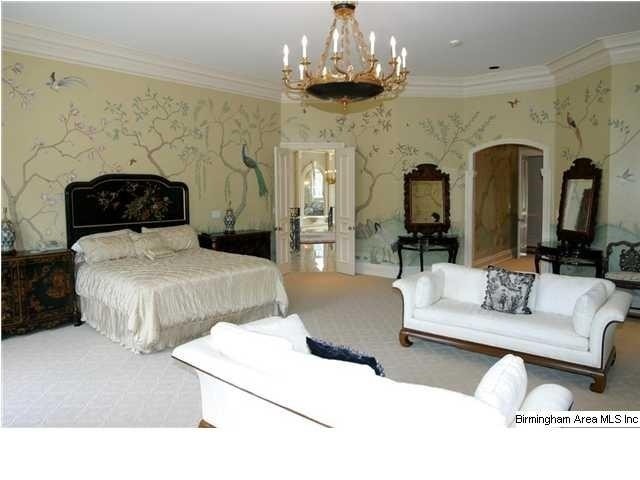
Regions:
[0,205,20,257]
[223,199,237,236]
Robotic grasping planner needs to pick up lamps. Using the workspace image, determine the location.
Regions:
[279,2,414,113]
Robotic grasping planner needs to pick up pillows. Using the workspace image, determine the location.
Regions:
[140,225,203,263]
[301,331,387,381]
[478,263,538,317]
[68,227,139,269]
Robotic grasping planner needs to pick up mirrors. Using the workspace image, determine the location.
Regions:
[302,161,327,218]
[403,163,451,236]
[555,156,603,247]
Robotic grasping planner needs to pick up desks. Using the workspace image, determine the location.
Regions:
[390,235,460,279]
[534,240,603,281]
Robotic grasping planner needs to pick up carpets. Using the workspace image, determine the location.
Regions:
[299,233,336,244]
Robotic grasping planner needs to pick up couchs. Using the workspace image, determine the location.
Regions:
[168,313,576,427]
[390,260,635,397]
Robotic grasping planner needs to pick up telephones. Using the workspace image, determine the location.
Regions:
[36,240,64,251]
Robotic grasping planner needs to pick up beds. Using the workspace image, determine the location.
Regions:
[62,172,279,355]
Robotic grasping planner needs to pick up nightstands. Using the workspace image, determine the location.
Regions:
[1,246,86,340]
[198,230,273,265]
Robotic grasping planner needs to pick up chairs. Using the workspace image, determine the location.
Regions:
[602,238,640,321]
